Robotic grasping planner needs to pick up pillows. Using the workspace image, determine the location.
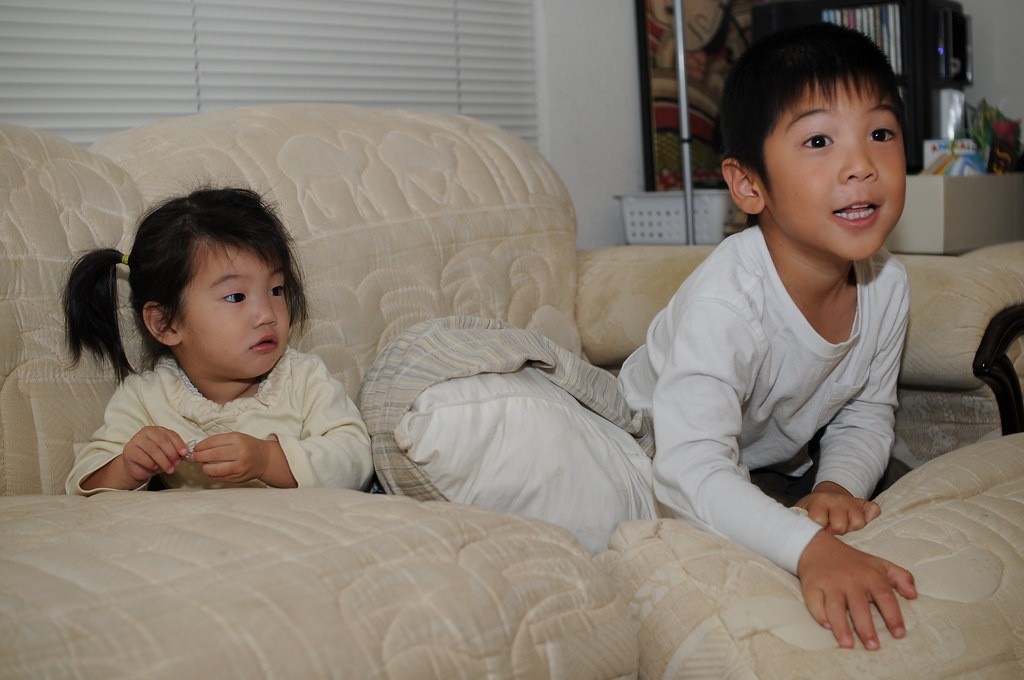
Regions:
[389,364,659,554]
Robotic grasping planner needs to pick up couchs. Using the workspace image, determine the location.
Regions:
[0,106,1024,680]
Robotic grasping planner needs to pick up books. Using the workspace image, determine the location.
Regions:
[822,4,903,98]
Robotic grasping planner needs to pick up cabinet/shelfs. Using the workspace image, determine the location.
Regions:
[801,0,966,177]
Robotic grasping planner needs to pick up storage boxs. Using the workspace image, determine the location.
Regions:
[613,189,741,244]
[880,174,1024,254]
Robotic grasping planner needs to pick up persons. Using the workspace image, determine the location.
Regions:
[60,189,376,496]
[619,21,917,651]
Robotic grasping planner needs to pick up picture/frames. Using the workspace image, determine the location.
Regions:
[634,0,759,185]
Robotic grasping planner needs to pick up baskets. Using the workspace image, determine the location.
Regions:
[615,189,733,246]
